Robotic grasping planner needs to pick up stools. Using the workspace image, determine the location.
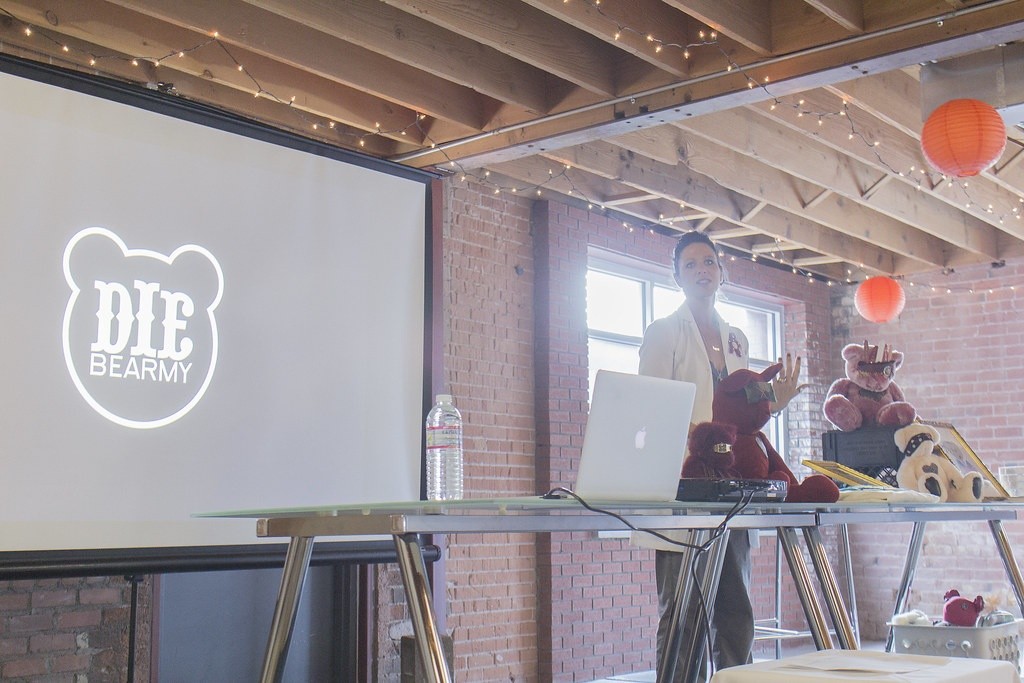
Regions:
[710,649,1021,683]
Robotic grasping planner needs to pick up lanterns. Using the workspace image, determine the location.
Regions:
[854,275,906,323]
[921,95,1007,179]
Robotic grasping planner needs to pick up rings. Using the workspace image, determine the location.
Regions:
[797,384,803,392]
[779,376,787,382]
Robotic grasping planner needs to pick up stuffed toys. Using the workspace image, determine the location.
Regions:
[680,418,740,481]
[893,421,982,504]
[823,340,918,432]
[888,589,1013,629]
[709,363,841,503]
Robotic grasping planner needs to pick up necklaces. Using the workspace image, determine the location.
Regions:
[698,320,721,352]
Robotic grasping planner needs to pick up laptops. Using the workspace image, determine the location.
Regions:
[519,370,697,502]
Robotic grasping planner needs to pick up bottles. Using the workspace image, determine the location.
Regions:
[425,394,464,501]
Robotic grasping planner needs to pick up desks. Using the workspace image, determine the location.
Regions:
[191,497,1023,683]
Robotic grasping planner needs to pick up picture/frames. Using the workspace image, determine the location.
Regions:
[920,423,1009,503]
[802,460,891,489]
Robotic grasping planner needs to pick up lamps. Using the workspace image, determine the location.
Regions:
[853,275,904,323]
[921,99,1006,177]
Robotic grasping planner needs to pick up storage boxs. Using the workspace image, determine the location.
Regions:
[887,616,1021,675]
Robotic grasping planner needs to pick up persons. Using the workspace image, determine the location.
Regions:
[638,230,813,683]
